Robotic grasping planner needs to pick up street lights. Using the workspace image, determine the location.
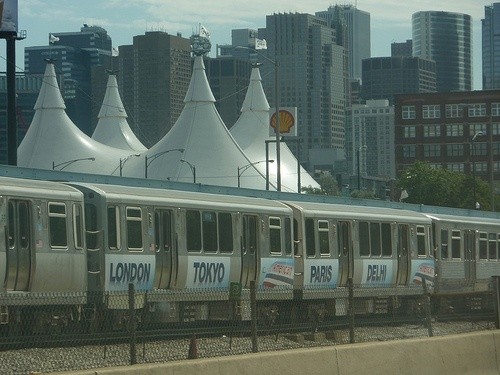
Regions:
[237,160,275,188]
[119,154,142,177]
[52,158,96,171]
[471,131,484,209]
[357,145,368,190]
[144,148,185,179]
[180,159,196,183]
[234,45,282,192]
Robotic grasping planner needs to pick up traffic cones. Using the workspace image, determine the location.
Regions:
[187,333,200,360]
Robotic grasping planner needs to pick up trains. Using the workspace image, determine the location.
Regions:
[0,163,500,340]
